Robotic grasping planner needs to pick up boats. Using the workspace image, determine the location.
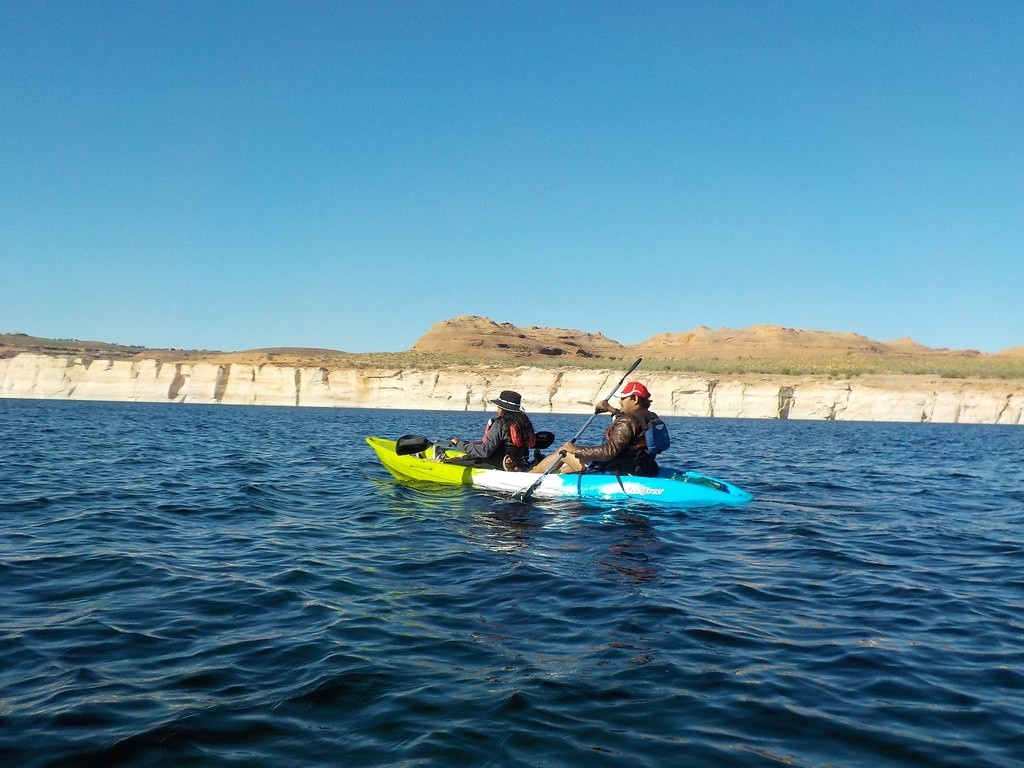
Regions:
[367,435,756,510]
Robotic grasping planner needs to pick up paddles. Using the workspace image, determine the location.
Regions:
[522,355,646,501]
[396,431,556,456]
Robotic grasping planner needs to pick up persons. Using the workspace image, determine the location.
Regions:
[503,382,658,476]
[416,390,534,468]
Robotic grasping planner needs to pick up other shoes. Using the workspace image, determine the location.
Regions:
[434,445,449,459]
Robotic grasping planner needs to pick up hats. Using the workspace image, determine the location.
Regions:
[614,382,651,398]
[487,390,525,413]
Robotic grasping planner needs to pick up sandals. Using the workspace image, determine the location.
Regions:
[533,449,546,464]
[503,455,520,473]
